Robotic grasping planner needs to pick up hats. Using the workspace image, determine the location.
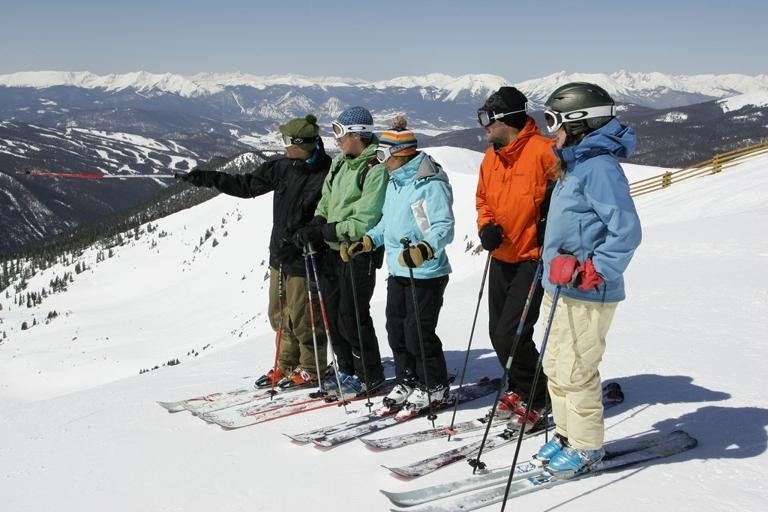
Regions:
[278,114,320,150]
[378,116,417,157]
[336,106,373,138]
[479,87,530,127]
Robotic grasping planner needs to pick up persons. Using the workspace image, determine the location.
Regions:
[291,106,389,399]
[476,86,558,434]
[338,116,456,411]
[174,115,332,386]
[537,81,642,475]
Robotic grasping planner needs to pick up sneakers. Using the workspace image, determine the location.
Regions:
[494,389,523,420]
[322,370,352,390]
[266,366,290,386]
[335,372,385,400]
[382,382,415,407]
[506,399,552,433]
[288,366,327,389]
[536,432,568,461]
[403,383,450,412]
[548,444,606,480]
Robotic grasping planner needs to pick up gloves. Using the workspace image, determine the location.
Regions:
[182,169,217,188]
[478,223,503,251]
[548,254,602,292]
[299,220,337,245]
[399,240,433,269]
[290,216,326,247]
[339,234,374,262]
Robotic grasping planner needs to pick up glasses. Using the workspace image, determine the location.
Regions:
[543,106,584,134]
[477,108,504,125]
[280,136,304,148]
[373,144,402,163]
[331,121,358,139]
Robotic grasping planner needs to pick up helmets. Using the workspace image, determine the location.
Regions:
[543,82,617,144]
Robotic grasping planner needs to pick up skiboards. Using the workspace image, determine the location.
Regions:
[352,381,624,477]
[380,430,698,512]
[159,367,334,418]
[199,376,399,429]
[159,367,696,512]
[280,376,506,449]
